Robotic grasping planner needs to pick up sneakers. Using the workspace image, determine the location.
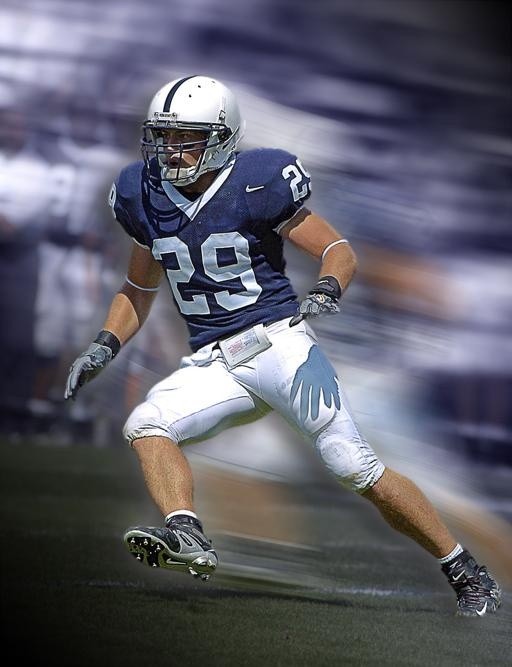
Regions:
[123,515,218,582]
[441,547,501,618]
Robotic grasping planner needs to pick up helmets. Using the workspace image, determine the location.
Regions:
[140,75,244,186]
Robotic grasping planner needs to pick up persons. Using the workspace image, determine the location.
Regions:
[62,72,506,620]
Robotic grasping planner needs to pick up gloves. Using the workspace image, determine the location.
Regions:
[289,276,341,328]
[64,331,120,400]
